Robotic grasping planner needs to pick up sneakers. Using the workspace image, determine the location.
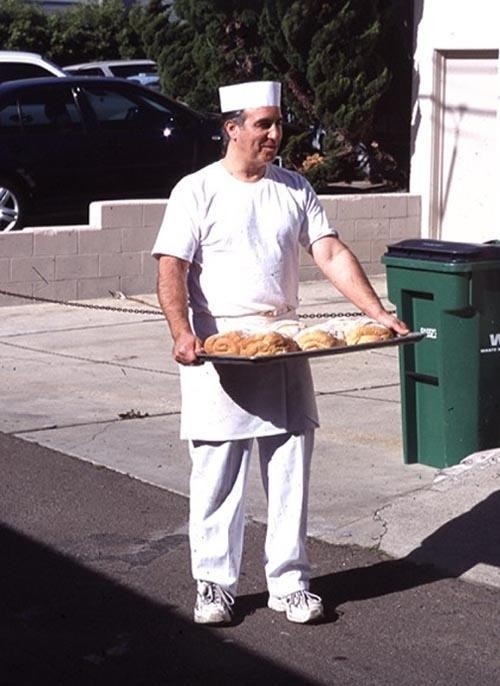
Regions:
[268,589,326,623]
[194,579,235,624]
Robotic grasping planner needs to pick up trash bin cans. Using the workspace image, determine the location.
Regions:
[380,238,500,469]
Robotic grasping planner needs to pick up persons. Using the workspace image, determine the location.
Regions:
[148,81,412,628]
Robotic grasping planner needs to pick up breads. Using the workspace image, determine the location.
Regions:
[203,317,395,356]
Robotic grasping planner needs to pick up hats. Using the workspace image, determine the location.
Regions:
[219,80,282,113]
